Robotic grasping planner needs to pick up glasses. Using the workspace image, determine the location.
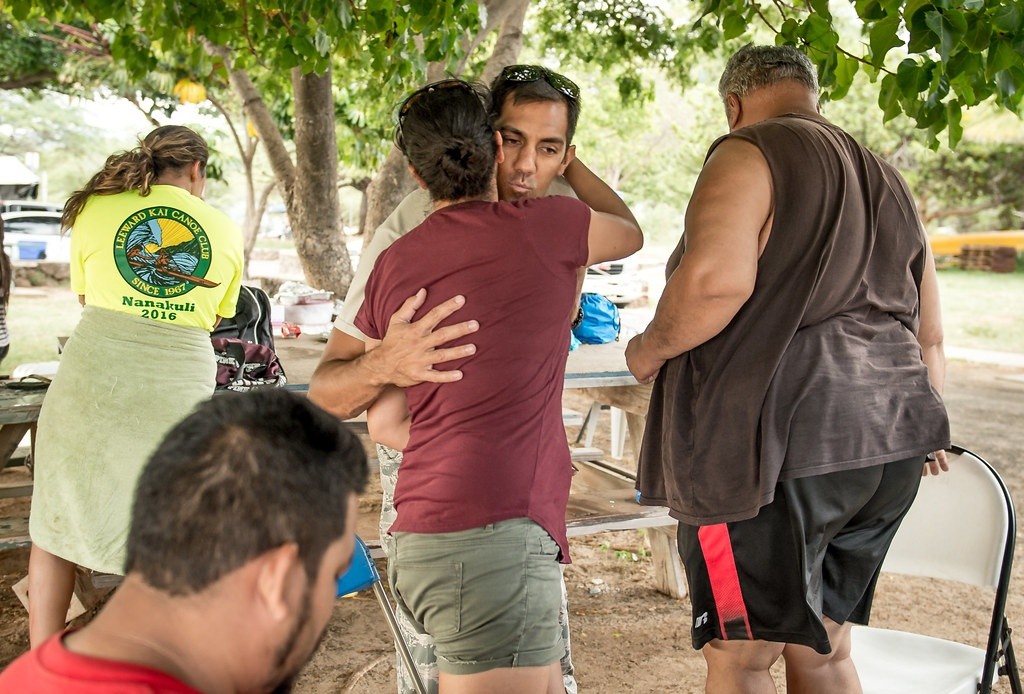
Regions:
[491,65,580,100]
[397,79,488,139]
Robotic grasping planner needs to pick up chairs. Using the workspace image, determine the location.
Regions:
[334,531,428,694]
[847,445,1022,694]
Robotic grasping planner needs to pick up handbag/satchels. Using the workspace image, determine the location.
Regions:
[210,337,286,392]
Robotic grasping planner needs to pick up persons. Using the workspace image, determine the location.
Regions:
[305,64,644,694]
[0,217,12,363]
[27,124,245,650]
[624,41,950,694]
[0,388,370,694]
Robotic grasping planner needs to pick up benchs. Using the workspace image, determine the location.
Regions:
[23,406,681,612]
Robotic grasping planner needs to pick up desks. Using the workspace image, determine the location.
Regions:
[0,339,688,601]
